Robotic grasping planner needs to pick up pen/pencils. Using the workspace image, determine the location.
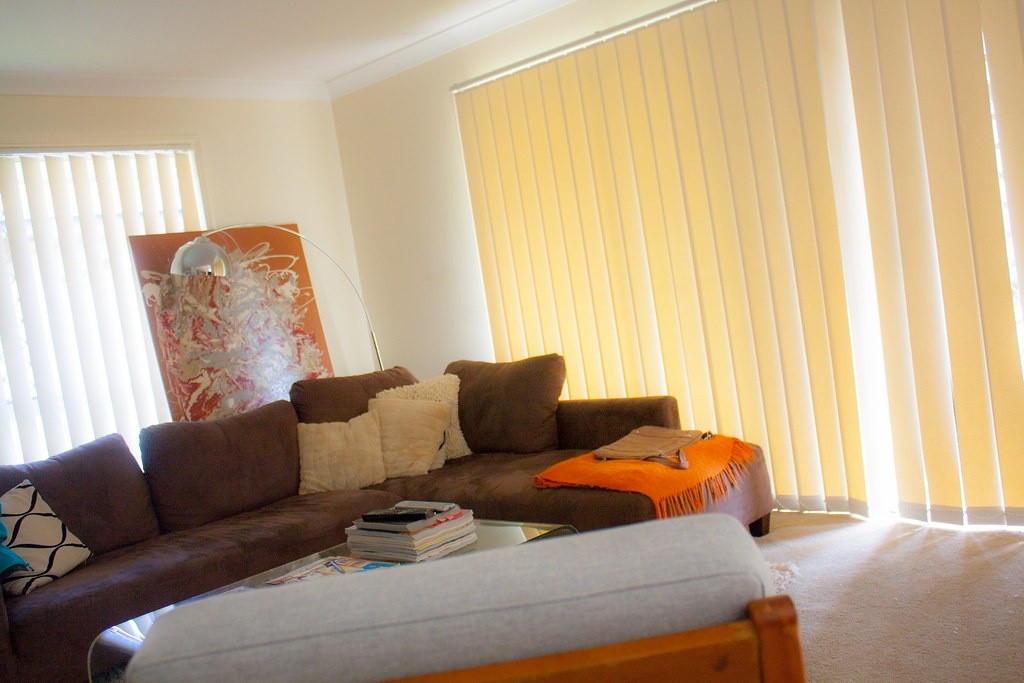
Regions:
[329,558,345,574]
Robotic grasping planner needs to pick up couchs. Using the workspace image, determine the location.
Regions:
[120,510,807,683]
[0,471,408,683]
[360,394,775,538]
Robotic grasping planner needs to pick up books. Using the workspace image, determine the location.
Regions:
[344,509,478,563]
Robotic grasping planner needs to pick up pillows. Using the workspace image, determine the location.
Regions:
[0,433,160,557]
[368,398,451,479]
[1,478,94,596]
[138,399,301,533]
[443,352,568,453]
[375,373,473,459]
[289,365,420,424]
[296,408,387,496]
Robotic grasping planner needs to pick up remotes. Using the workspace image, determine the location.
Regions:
[362,508,434,523]
[395,500,456,512]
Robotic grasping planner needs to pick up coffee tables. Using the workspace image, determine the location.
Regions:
[86,518,581,683]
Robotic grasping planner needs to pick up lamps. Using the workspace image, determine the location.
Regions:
[169,223,385,372]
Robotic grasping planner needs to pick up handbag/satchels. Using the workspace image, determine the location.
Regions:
[592,426,713,469]
